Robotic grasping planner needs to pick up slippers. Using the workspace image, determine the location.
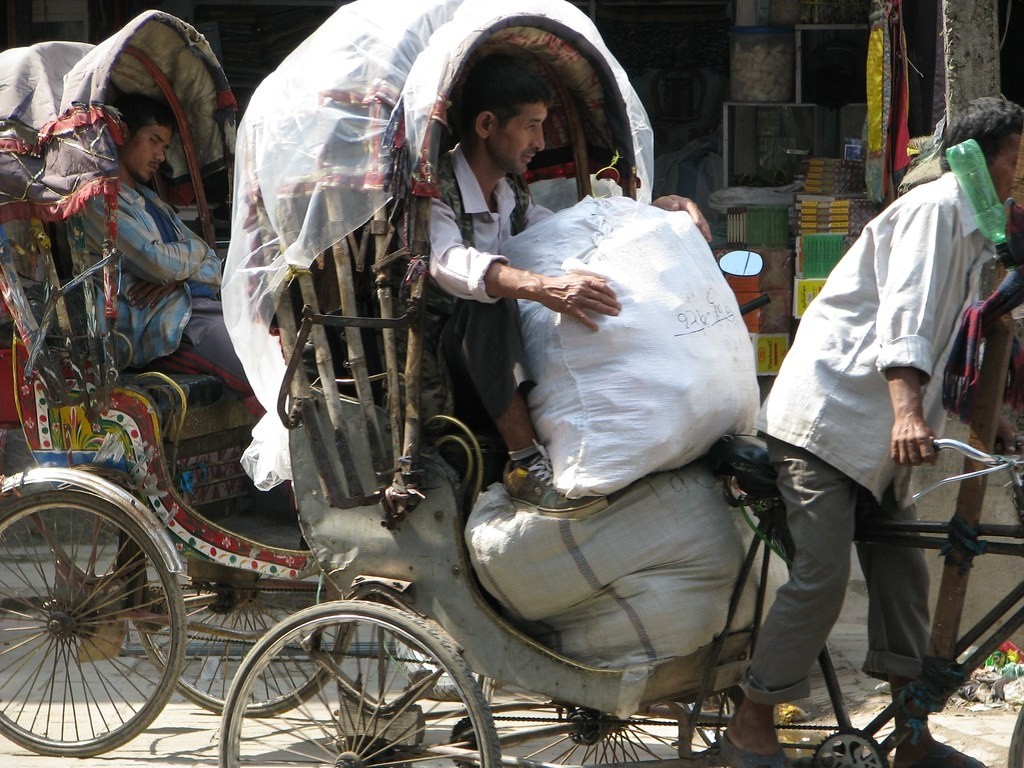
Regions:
[717,729,834,768]
[890,741,987,768]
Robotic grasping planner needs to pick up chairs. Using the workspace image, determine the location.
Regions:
[0,201,298,506]
[333,372,509,462]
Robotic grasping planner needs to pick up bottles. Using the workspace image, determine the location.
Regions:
[946,138,1007,243]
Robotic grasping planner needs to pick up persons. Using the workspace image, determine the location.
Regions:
[720,96,1024,768]
[424,52,711,520]
[92,93,310,552]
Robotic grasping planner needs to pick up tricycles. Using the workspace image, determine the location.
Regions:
[216,0,1024,768]
[0,0,361,765]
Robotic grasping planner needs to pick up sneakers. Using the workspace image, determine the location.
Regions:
[503,438,609,517]
